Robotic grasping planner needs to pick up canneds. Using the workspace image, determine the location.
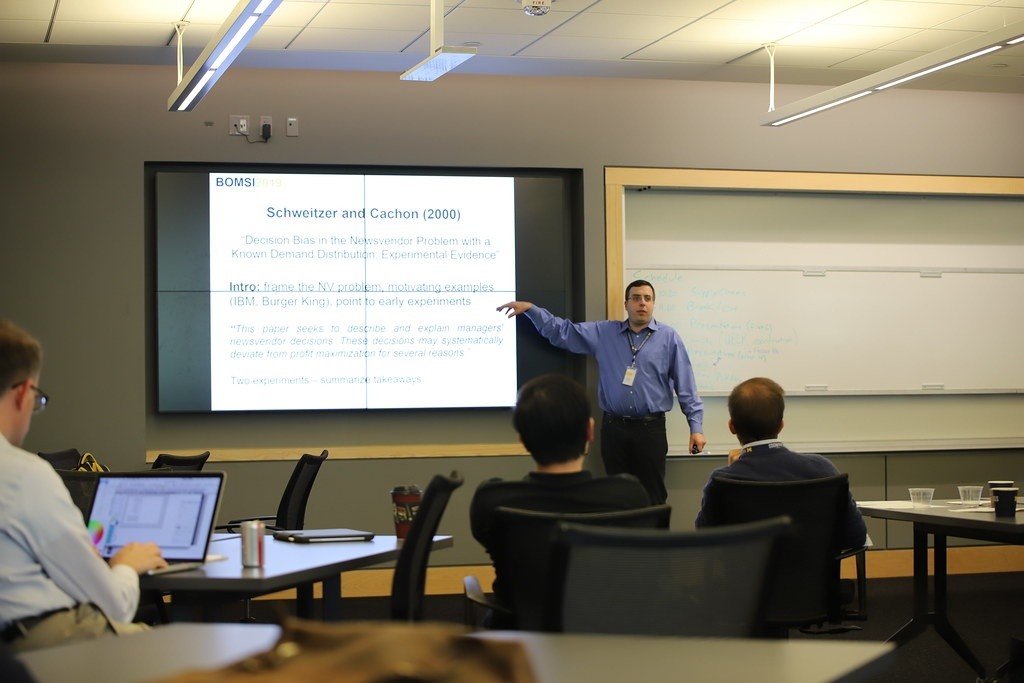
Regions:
[241,520,265,567]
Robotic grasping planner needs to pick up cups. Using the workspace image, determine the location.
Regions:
[908,488,935,512]
[988,480,1014,507]
[958,485,983,509]
[390,483,424,541]
[991,487,1019,519]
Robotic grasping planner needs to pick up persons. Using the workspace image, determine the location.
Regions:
[496,280,707,507]
[0,317,170,661]
[470,376,655,633]
[695,378,867,550]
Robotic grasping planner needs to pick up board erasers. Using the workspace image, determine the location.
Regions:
[921,384,944,390]
[804,385,828,392]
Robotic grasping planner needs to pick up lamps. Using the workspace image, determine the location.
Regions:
[167,0,280,113]
[758,20,1024,128]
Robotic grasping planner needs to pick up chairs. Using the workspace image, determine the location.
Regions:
[33,442,869,643]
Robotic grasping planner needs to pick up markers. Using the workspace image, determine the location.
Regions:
[692,444,699,454]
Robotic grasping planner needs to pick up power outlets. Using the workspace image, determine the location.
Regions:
[229,113,249,135]
[260,116,272,136]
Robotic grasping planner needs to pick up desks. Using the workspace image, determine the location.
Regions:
[14,627,901,682]
[135,521,454,595]
[850,493,1024,683]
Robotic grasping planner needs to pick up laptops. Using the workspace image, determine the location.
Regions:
[274,528,374,543]
[86,471,228,576]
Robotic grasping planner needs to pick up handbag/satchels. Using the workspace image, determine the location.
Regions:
[71,452,110,472]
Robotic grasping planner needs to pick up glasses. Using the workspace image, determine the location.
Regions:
[12,381,50,415]
[628,295,651,302]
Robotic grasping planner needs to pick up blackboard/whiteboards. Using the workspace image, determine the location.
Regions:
[603,166,1024,399]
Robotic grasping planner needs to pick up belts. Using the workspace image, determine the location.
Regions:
[604,412,657,422]
[0,609,70,643]
